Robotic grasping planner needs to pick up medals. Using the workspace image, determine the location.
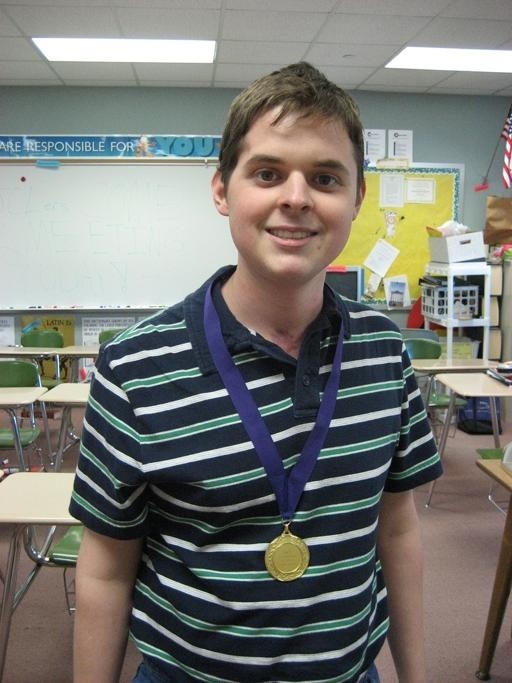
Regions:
[262,523,311,583]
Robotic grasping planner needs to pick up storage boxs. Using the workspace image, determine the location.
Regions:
[429,230,486,264]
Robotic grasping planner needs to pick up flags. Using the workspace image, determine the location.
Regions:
[501,105,512,189]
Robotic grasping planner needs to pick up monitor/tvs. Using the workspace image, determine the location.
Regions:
[325,266,361,302]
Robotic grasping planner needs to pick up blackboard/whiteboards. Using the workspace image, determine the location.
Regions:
[1,158,466,313]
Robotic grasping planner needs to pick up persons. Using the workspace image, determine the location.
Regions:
[69,60,445,680]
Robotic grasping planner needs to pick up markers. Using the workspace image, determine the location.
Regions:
[27,305,167,309]
